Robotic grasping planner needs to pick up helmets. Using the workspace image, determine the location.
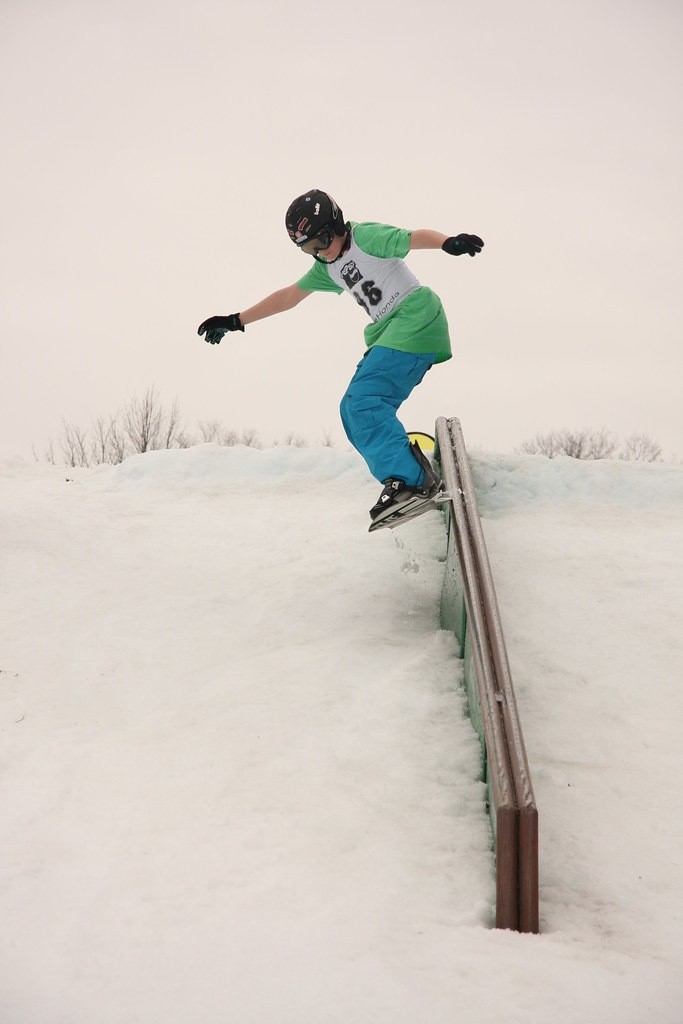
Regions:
[285,189,345,248]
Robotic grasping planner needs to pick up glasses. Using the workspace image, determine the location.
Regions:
[300,226,335,256]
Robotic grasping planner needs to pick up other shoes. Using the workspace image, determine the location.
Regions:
[369,441,443,532]
[385,439,453,529]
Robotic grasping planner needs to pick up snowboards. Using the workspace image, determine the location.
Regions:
[368,497,446,534]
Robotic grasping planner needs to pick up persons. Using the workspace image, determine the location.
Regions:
[198,190,485,528]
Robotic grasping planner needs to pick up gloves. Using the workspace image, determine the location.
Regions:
[442,233,484,257]
[198,312,244,345]
